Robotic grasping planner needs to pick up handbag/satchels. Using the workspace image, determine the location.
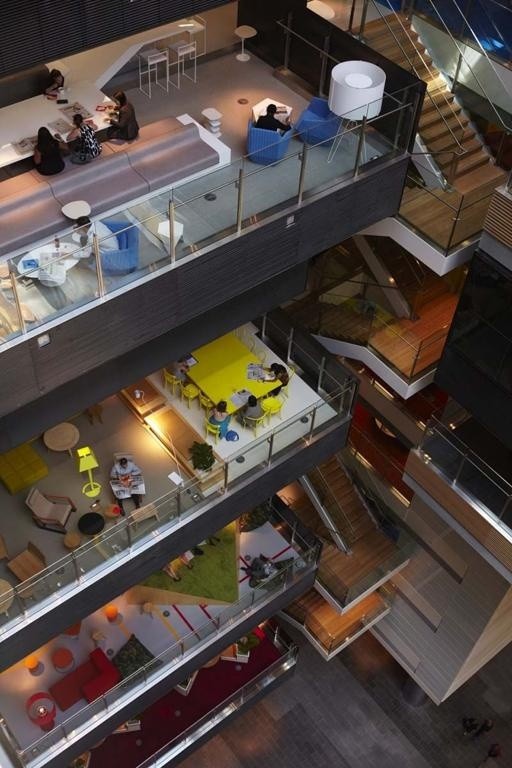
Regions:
[225,430,239,442]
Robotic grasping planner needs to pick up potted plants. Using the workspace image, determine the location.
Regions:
[189,441,215,471]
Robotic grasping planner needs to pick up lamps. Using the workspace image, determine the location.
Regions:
[26,692,56,730]
[326,60,387,164]
[75,448,103,498]
[136,390,186,485]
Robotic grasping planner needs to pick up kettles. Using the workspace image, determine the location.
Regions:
[134,390,145,400]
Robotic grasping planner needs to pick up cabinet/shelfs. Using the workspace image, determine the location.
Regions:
[120,378,165,418]
[146,405,227,496]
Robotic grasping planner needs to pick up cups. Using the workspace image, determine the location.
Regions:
[92,503,98,508]
[58,87,65,94]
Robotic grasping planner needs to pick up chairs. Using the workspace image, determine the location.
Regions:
[8,542,50,583]
[163,370,185,398]
[181,384,200,409]
[203,418,220,444]
[242,409,272,439]
[200,394,211,411]
[24,486,75,534]
[260,396,284,424]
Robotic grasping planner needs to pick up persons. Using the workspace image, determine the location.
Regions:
[61,216,120,257]
[236,395,263,426]
[42,68,65,95]
[157,533,222,582]
[241,561,285,583]
[262,362,290,396]
[34,126,69,176]
[174,358,190,387]
[110,457,144,517]
[209,401,232,440]
[255,104,292,132]
[66,114,103,165]
[103,88,140,140]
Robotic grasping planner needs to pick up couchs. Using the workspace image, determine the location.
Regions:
[49,648,119,711]
[245,122,293,166]
[91,222,140,273]
[296,97,341,147]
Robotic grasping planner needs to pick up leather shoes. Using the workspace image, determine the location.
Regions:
[119,506,125,516]
[135,504,140,508]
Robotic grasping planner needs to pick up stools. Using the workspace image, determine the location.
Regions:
[236,26,256,61]
[139,49,169,100]
[1,172,73,258]
[45,144,151,215]
[171,40,198,89]
[122,114,232,192]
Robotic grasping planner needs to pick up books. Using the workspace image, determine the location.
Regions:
[110,474,147,500]
[39,251,67,283]
[247,363,265,381]
[271,106,287,113]
[183,354,198,369]
[230,388,254,408]
[46,118,77,136]
[11,135,43,156]
[21,259,40,270]
[58,101,94,121]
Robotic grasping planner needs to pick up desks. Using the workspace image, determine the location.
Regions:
[79,512,104,536]
[185,333,282,413]
[0,579,13,615]
[42,422,79,457]
[0,82,119,169]
